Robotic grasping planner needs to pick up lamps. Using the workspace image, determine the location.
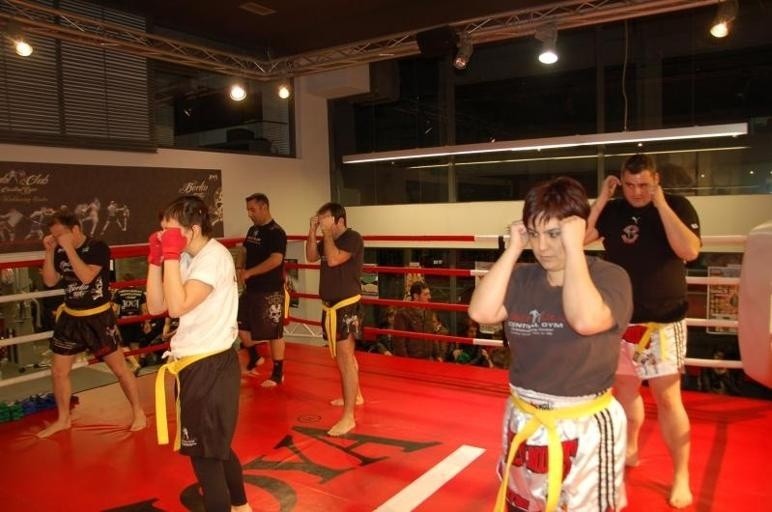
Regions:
[453,23,559,70]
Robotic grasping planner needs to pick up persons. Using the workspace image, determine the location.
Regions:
[236,193,289,389]
[432,310,450,363]
[452,317,482,366]
[466,172,635,512]
[374,304,398,355]
[707,341,736,394]
[137,315,180,369]
[118,202,131,231]
[100,200,122,236]
[24,205,46,241]
[35,208,150,439]
[143,195,254,512]
[305,201,365,438]
[111,268,154,377]
[583,151,705,509]
[391,280,446,363]
[476,330,505,369]
[0,207,24,245]
[81,198,100,238]
